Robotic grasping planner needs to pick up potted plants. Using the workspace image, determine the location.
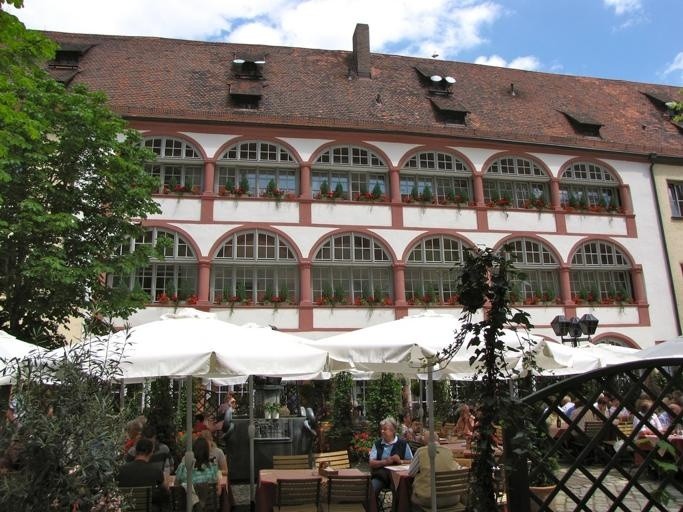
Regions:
[263,400,281,420]
[316,179,385,200]
[508,288,633,307]
[406,183,470,205]
[492,190,551,209]
[159,280,461,308]
[565,190,619,215]
[156,176,202,195]
[217,176,284,199]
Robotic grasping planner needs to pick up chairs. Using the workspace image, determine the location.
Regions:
[272,417,502,512]
[546,417,682,476]
[108,449,229,512]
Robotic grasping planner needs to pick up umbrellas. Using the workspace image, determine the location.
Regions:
[0,328,50,387]
[31,305,355,512]
[316,310,601,511]
[201,321,337,500]
[539,342,643,378]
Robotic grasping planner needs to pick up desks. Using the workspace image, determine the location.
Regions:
[253,436,293,457]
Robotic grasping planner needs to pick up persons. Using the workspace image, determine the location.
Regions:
[117,384,240,512]
[397,402,503,459]
[407,431,462,508]
[5,383,33,445]
[541,387,683,479]
[367,416,413,511]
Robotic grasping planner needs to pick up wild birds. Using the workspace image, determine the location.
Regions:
[432,53,439,60]
